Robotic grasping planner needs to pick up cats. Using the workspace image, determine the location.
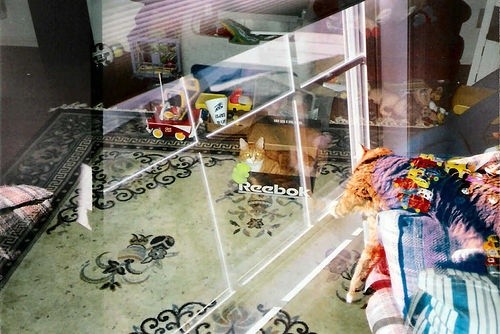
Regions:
[239,137,317,177]
[325,143,487,303]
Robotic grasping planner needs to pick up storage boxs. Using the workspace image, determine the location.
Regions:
[235,121,322,196]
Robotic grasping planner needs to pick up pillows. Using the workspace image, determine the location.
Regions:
[375,208,447,322]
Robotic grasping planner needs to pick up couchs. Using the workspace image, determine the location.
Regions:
[363,90,500,333]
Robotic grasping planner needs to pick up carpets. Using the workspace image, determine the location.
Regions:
[1,101,424,332]
